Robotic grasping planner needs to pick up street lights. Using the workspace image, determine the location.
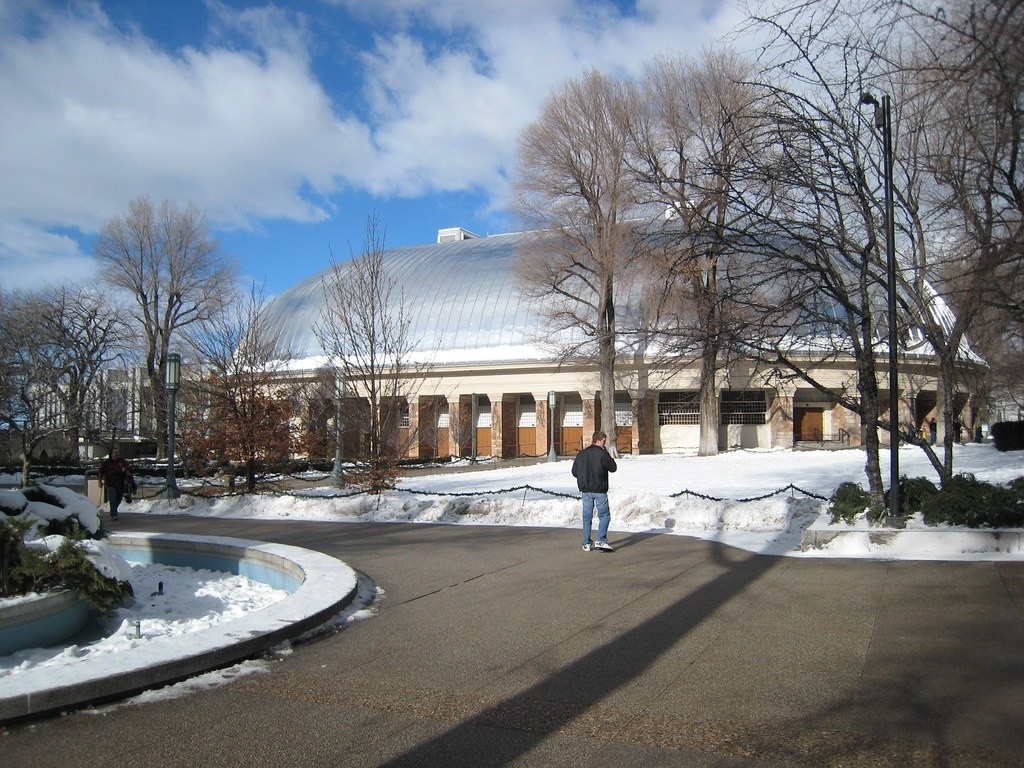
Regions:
[860,92,900,517]
[162,353,181,500]
[330,366,345,488]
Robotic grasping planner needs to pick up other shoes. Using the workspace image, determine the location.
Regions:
[113,515,118,520]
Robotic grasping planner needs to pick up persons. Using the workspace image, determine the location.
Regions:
[915,420,926,441]
[954,419,961,442]
[975,427,983,443]
[929,418,937,443]
[572,431,617,552]
[98,448,129,520]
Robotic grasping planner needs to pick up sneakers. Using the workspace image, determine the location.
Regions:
[582,544,591,551]
[594,540,613,551]
